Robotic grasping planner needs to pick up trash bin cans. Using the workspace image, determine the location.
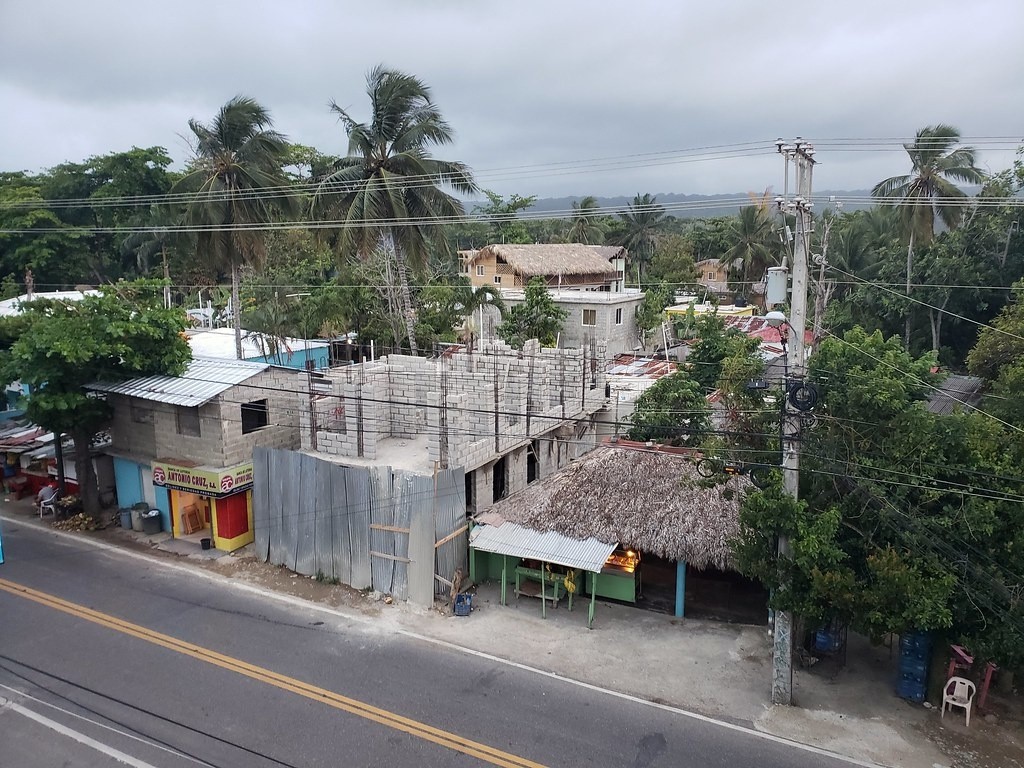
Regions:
[454,594,472,616]
[130,502,148,531]
[120,508,132,530]
[141,509,163,535]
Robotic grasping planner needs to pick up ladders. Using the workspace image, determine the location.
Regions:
[663,320,675,347]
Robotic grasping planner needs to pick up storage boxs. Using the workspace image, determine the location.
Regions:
[896,633,933,705]
[454,594,472,617]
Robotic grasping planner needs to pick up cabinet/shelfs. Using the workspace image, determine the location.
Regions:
[807,618,848,678]
[516,561,578,609]
[896,635,932,702]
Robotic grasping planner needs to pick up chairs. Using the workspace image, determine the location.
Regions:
[941,676,976,727]
[39,488,62,518]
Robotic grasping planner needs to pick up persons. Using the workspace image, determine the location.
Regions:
[3,459,15,494]
[35,482,53,515]
